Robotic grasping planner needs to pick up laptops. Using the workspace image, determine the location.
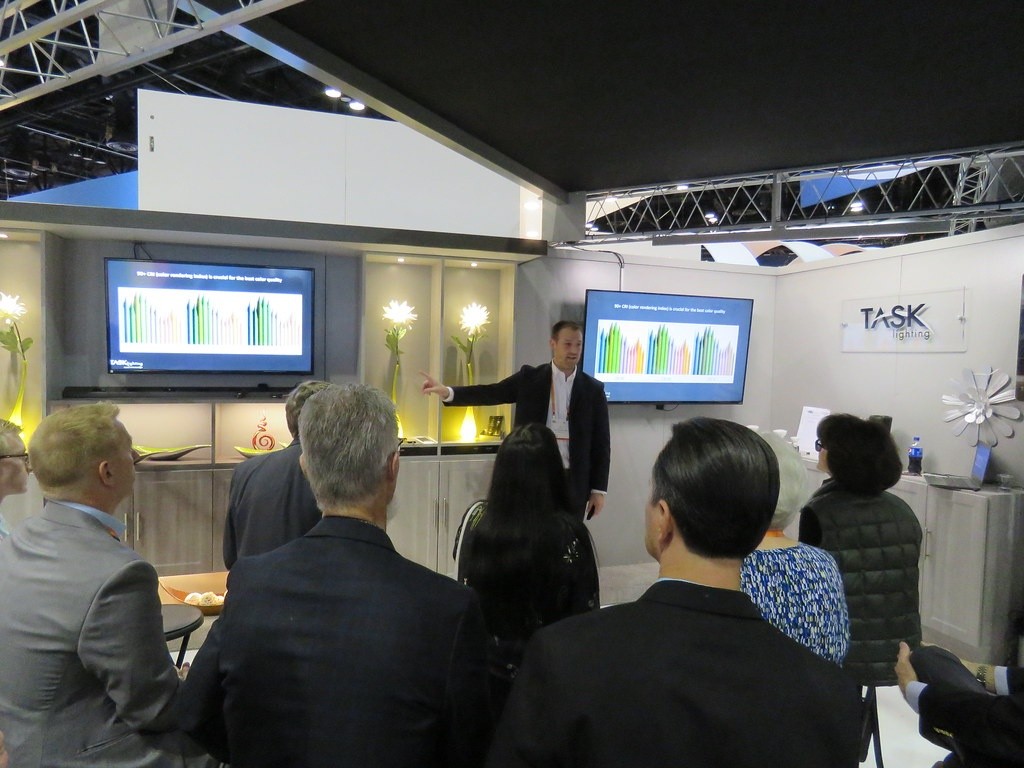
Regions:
[925,439,992,490]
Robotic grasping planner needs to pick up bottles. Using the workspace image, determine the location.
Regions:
[908,437,923,473]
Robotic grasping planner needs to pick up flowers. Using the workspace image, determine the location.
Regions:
[449,302,492,365]
[382,300,417,364]
[0,292,34,360]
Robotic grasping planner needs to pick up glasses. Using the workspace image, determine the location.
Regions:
[394,438,403,453]
[815,439,825,452]
[0,453,29,465]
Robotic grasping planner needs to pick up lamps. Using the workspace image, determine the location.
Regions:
[104,107,138,154]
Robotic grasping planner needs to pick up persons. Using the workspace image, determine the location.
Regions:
[180,381,503,767]
[891,640,1024,768]
[0,401,178,767]
[419,320,610,523]
[733,411,924,688]
[452,425,597,694]
[511,417,861,768]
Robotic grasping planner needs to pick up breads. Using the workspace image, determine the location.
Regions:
[184,591,224,606]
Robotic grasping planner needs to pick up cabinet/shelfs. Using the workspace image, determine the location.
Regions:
[893,481,1023,670]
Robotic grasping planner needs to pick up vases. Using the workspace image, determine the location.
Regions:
[9,360,27,430]
[461,360,477,444]
[391,363,402,439]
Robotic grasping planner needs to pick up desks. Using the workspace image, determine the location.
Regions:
[161,603,204,669]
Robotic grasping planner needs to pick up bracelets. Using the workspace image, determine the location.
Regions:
[974,663,986,690]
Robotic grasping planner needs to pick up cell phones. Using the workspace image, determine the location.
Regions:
[587,504,594,520]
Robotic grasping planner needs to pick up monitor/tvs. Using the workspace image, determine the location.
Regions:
[103,257,315,375]
[581,289,754,406]
